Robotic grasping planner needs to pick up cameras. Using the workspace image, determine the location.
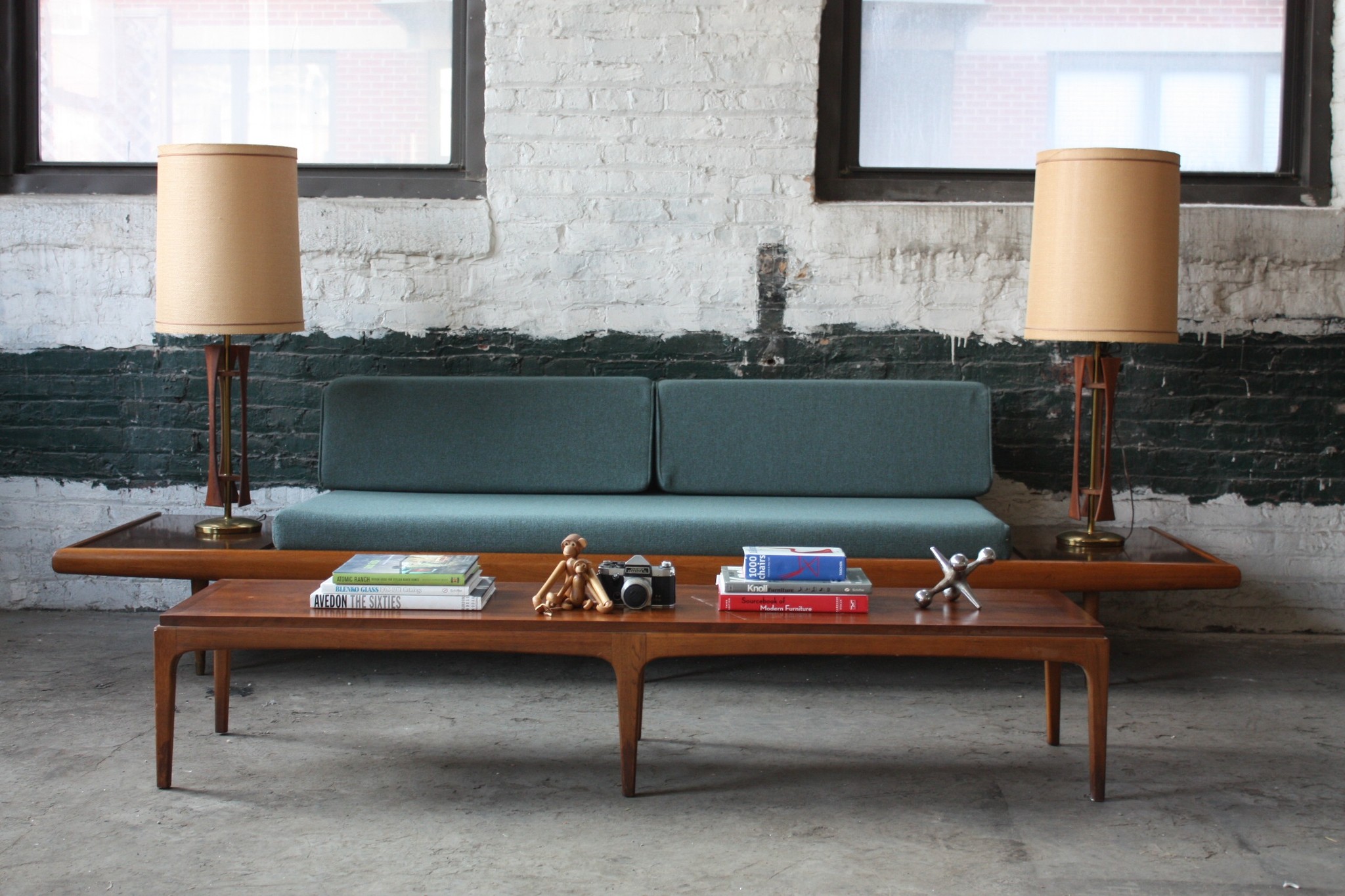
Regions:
[595,555,676,610]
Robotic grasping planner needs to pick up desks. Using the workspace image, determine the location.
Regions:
[152,578,1112,803]
[55,512,1244,674]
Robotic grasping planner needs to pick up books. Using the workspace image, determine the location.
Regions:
[716,545,872,614]
[310,554,498,611]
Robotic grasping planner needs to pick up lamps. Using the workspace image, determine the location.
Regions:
[1023,149,1183,550]
[155,144,303,536]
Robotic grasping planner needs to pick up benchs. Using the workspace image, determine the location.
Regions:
[271,373,1008,560]
